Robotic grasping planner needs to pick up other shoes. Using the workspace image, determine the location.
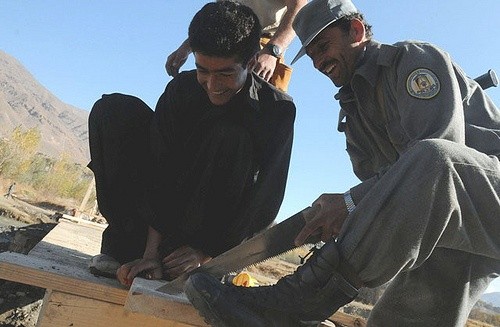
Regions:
[88,254,120,278]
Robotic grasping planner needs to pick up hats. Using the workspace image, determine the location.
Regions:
[290,0,358,65]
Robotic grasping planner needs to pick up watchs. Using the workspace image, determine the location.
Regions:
[265,44,281,57]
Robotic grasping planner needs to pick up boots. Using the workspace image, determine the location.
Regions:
[186,236,359,327]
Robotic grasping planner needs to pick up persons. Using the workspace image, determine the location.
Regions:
[87,2,297,286]
[183,0,500,327]
[165,0,312,92]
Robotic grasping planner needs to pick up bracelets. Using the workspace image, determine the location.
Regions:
[343,189,356,212]
